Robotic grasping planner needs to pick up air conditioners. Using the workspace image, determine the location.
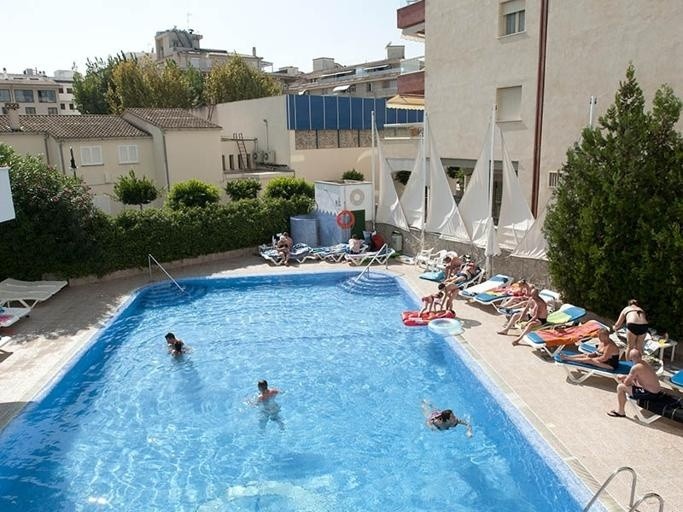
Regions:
[251,149,274,165]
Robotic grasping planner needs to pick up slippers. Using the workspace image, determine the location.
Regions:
[607,410,627,417]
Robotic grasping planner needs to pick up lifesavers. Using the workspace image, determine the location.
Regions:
[289,242,309,258]
[349,189,365,205]
[336,209,355,229]
[428,318,461,334]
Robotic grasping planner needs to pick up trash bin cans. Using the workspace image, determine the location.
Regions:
[391,231,404,251]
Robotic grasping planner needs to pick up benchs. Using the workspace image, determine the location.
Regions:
[451,265,682,425]
[0,277,67,328]
[257,242,394,265]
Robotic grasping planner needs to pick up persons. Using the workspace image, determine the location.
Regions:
[421,398,473,438]
[612,299,649,361]
[164,333,188,348]
[559,328,620,370]
[608,348,663,418]
[245,379,285,432]
[419,252,546,346]
[168,341,186,356]
[273,231,294,266]
[348,233,361,254]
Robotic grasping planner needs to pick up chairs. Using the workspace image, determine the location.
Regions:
[413,247,458,273]
